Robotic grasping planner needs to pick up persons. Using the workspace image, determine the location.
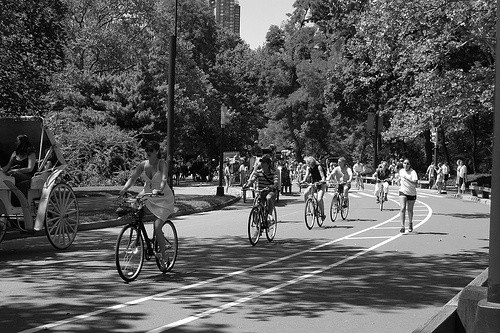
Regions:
[242,156,279,236]
[299,156,326,221]
[0,134,37,207]
[326,157,353,213]
[174,151,403,204]
[394,158,418,234]
[118,142,174,271]
[426,160,468,195]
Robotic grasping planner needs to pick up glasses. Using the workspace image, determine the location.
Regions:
[144,148,154,153]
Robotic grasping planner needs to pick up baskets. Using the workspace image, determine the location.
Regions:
[113,194,143,225]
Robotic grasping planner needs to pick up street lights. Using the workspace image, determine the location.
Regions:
[216,102,229,195]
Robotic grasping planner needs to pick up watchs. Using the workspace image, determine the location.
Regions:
[160,188,163,191]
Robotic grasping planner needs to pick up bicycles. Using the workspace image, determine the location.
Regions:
[113,192,178,282]
[354,172,364,191]
[300,182,323,230]
[328,182,350,222]
[241,187,279,246]
[376,178,388,211]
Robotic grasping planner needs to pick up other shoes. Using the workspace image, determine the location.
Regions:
[341,198,348,206]
[400,228,405,233]
[408,224,412,232]
[268,214,274,222]
[130,240,141,248]
[159,255,169,271]
[252,230,258,239]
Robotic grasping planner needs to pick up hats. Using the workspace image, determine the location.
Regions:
[259,157,272,165]
[144,142,160,150]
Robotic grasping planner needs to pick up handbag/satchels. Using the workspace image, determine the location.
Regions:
[461,182,466,190]
